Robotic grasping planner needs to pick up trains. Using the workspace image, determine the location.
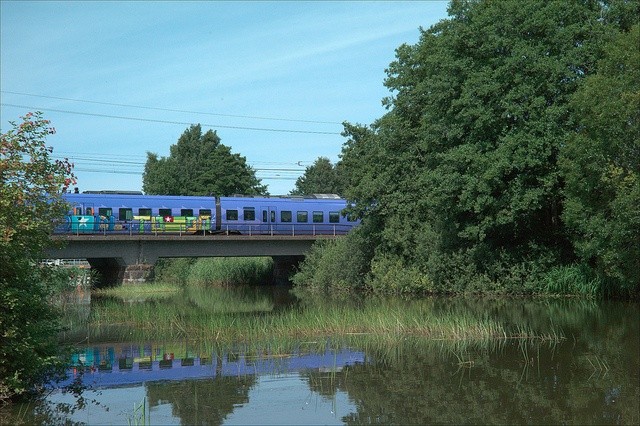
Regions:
[26,191,367,235]
[41,341,371,387]
